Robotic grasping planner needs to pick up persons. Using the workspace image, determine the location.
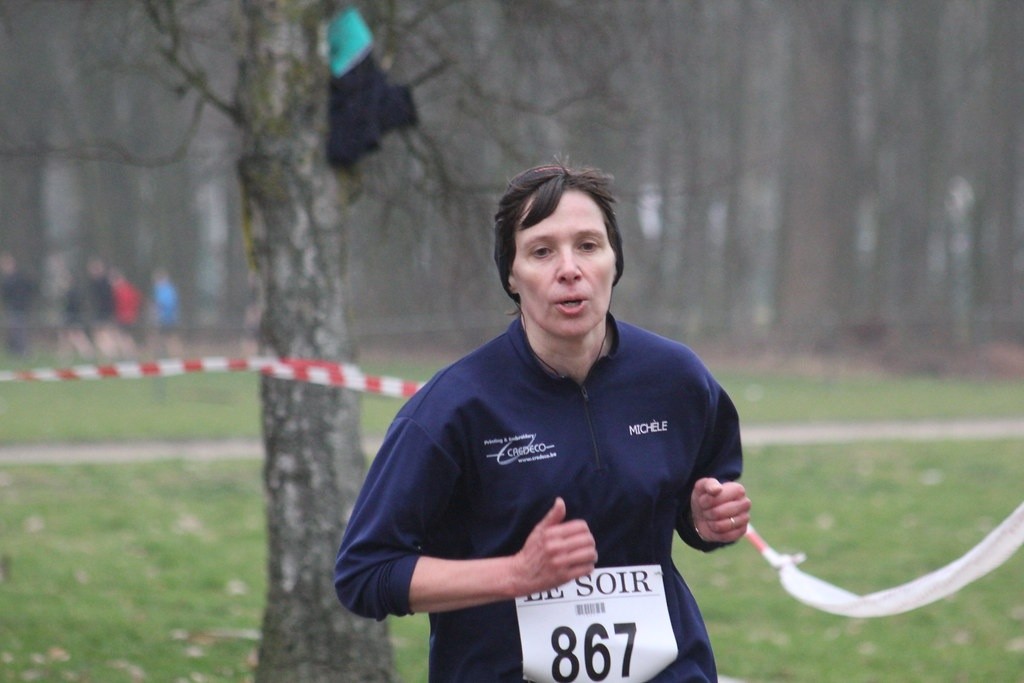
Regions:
[332,167,753,683]
[0,244,178,368]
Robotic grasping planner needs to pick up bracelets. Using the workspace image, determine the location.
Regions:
[694,523,703,539]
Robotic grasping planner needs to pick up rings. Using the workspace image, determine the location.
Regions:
[730,517,735,529]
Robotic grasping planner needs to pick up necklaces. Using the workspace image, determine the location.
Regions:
[531,327,609,384]
